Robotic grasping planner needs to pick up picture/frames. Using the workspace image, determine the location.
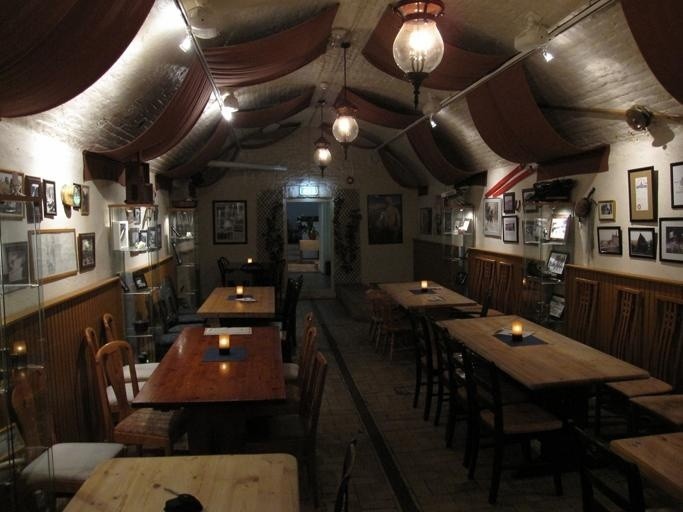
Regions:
[212,198,247,244]
[367,193,404,245]
[0,165,97,299]
[416,160,681,264]
[124,204,190,291]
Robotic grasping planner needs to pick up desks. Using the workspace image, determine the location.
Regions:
[131,327,286,436]
[59,451,305,511]
[299,239,319,259]
[609,431,682,502]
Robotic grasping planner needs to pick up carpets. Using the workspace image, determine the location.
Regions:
[284,287,337,302]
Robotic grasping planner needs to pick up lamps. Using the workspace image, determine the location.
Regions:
[330,41,360,161]
[222,86,241,113]
[427,40,554,131]
[390,1,446,115]
[311,100,333,181]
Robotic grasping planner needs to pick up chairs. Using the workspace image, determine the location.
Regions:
[147,255,309,327]
[331,440,358,510]
[5,362,128,510]
[81,313,183,453]
[451,254,683,431]
[247,311,328,457]
[362,279,479,451]
[463,343,564,505]
[569,417,646,510]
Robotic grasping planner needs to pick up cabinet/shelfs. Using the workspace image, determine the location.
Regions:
[106,199,162,364]
[1,198,59,512]
[166,205,200,313]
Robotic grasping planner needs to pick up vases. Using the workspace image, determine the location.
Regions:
[307,228,316,240]
